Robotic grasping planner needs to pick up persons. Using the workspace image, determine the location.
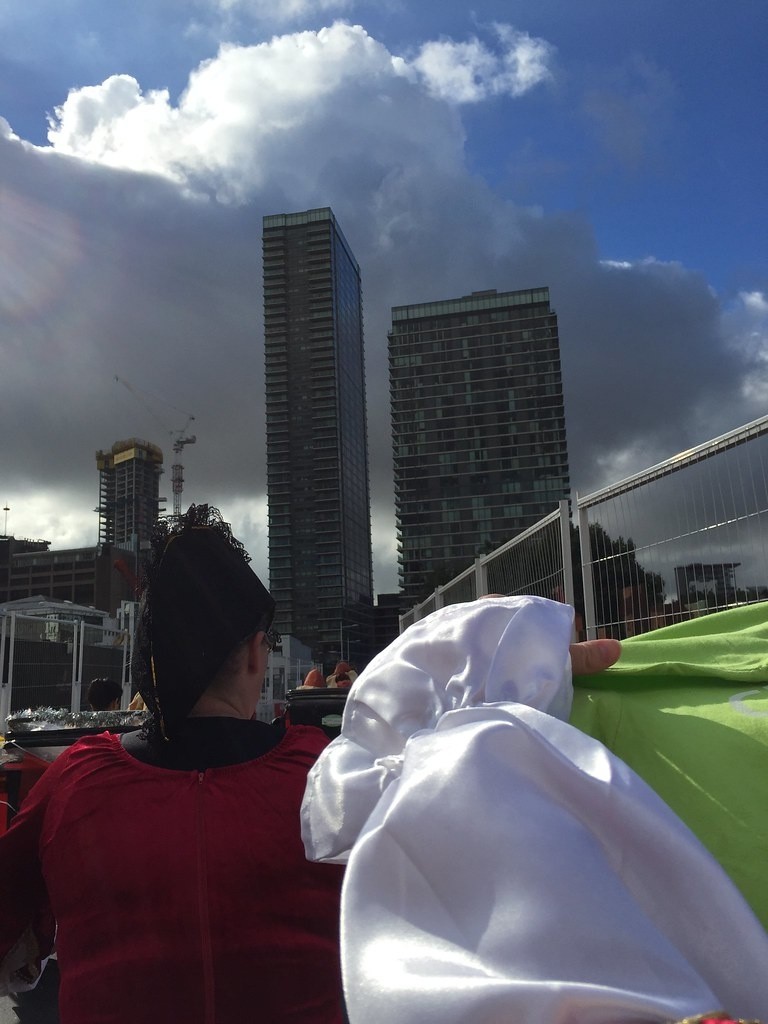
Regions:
[0,524,768,1024]
[87,678,123,712]
